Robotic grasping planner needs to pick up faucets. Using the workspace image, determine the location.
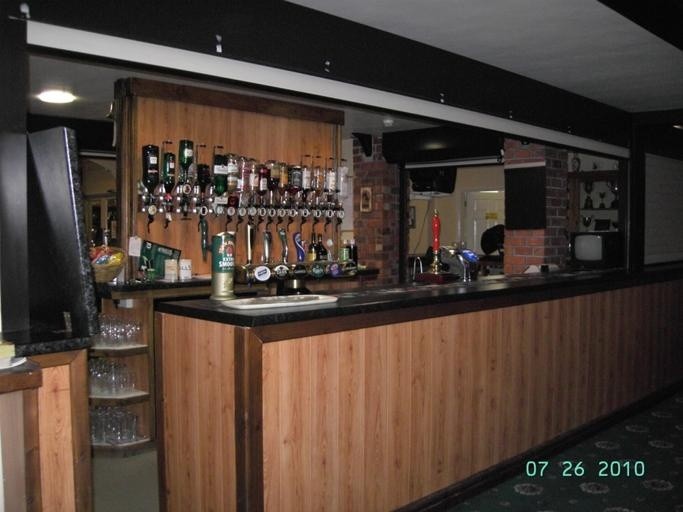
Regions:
[412,257,423,281]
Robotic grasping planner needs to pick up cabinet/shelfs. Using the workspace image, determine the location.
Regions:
[570,169,628,272]
[128,76,346,284]
[87,300,164,512]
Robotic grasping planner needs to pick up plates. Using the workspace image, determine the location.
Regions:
[222,295,337,310]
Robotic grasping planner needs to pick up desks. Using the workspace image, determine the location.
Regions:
[151,269,602,512]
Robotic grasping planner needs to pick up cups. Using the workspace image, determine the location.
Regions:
[90,357,135,395]
[91,407,139,443]
[95,313,142,344]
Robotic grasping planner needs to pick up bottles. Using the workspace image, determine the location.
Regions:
[235,156,251,203]
[286,164,302,203]
[336,166,350,204]
[212,154,228,198]
[300,166,312,203]
[196,163,210,198]
[247,158,260,205]
[339,239,350,261]
[265,159,280,203]
[324,168,337,203]
[312,166,324,203]
[225,153,240,195]
[317,234,328,267]
[275,162,288,204]
[178,139,195,181]
[162,152,177,194]
[257,165,269,205]
[142,145,160,193]
[349,239,358,265]
[306,232,317,271]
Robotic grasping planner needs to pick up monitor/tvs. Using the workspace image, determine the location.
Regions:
[571,233,612,268]
